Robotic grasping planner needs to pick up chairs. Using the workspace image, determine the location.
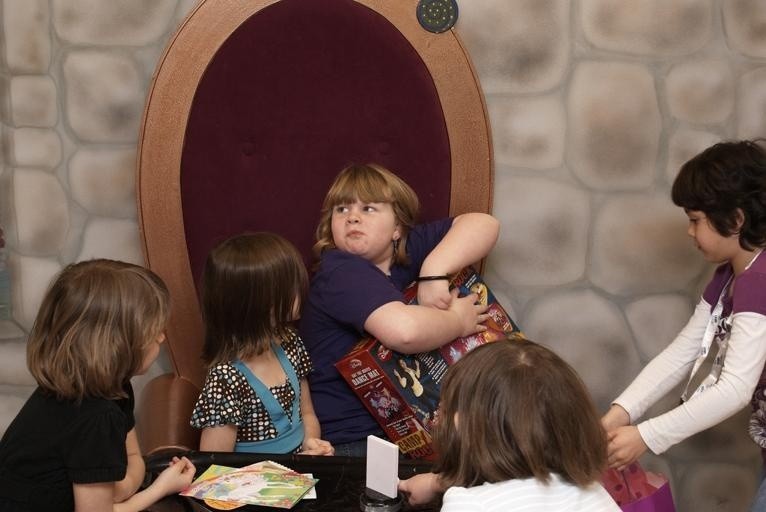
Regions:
[134,1,494,458]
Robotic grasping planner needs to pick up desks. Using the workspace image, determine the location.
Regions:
[138,443,487,511]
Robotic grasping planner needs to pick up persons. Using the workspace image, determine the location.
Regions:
[596,139,766,479]
[299,162,500,459]
[392,338,627,511]
[189,231,337,456]
[0,257,196,512]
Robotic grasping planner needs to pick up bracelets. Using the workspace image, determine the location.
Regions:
[416,274,449,280]
